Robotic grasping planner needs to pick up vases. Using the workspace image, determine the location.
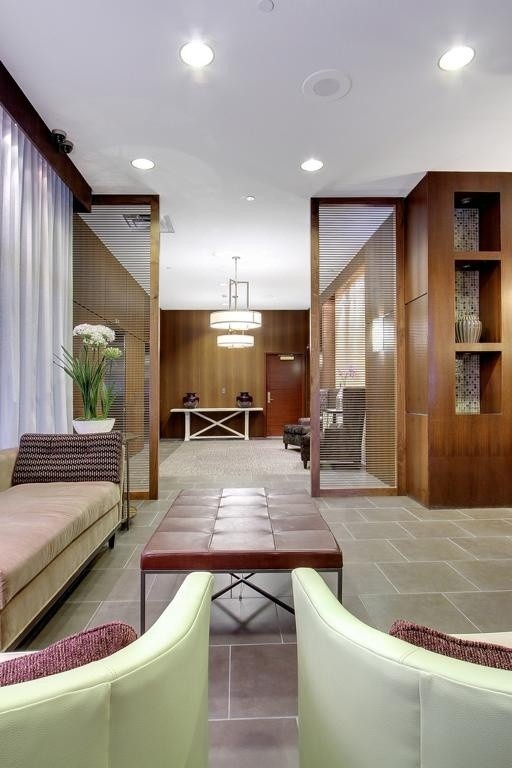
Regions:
[236,391,253,408]
[455,310,483,342]
[183,393,199,408]
[71,417,116,435]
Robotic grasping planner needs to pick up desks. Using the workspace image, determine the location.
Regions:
[120,432,139,531]
[169,407,264,441]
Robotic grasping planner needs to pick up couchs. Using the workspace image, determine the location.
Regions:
[0,432,125,653]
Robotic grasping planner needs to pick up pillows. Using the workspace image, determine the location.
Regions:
[387,619,512,671]
[0,619,138,688]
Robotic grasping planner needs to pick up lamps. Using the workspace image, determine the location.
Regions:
[217,330,255,349]
[209,257,262,331]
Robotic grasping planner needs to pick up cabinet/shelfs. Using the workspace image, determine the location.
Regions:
[455,192,502,415]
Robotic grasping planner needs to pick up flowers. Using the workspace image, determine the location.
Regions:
[337,365,358,386]
[53,323,123,419]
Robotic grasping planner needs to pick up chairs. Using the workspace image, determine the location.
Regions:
[283,389,329,449]
[0,571,216,768]
[301,387,365,469]
[290,566,512,768]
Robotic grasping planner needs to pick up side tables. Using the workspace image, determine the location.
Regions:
[323,409,343,431]
